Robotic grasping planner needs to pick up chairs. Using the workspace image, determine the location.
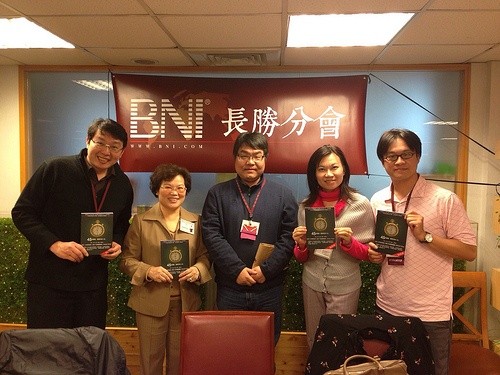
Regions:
[306,312,434,375]
[180,311,274,375]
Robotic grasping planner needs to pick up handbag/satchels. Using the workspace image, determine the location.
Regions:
[322,354,409,375]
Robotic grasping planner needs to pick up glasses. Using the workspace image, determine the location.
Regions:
[160,184,188,193]
[383,151,416,162]
[91,138,123,153]
[237,153,266,162]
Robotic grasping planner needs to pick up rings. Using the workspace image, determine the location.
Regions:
[190,276,193,280]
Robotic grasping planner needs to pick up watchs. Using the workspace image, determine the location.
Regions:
[419,230,433,244]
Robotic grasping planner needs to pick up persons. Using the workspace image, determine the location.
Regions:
[202,132,299,346]
[292,144,376,354]
[368,129,477,375]
[119,164,213,375]
[11,117,134,330]
